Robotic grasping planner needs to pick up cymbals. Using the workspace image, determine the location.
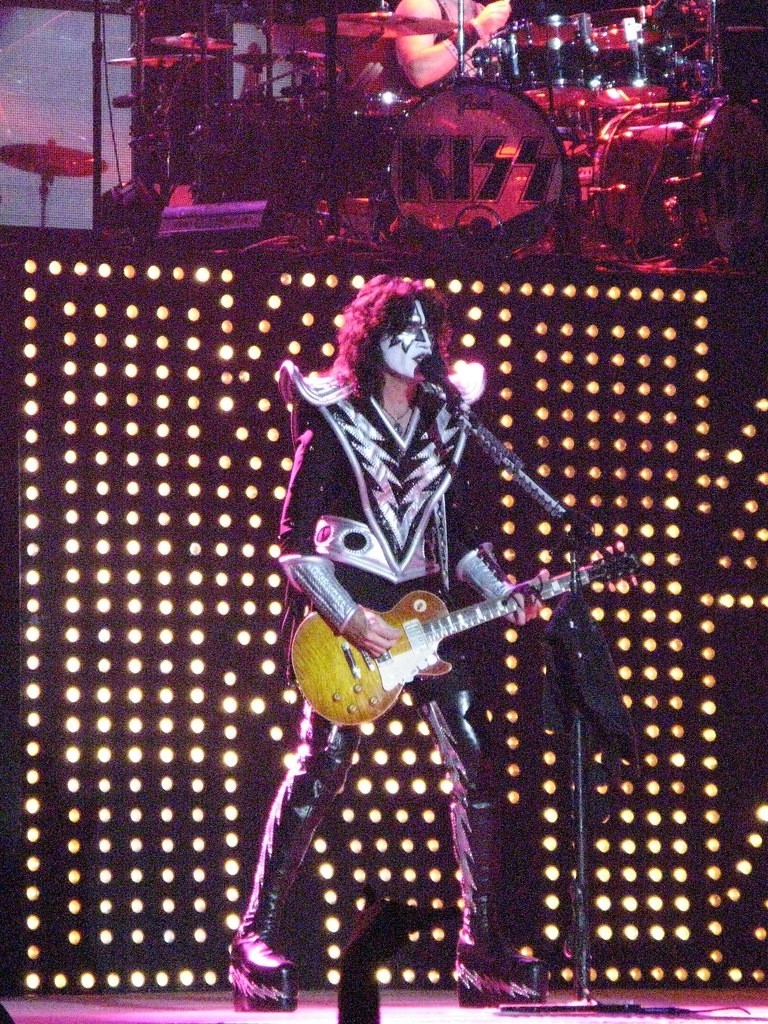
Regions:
[148,32,239,51]
[234,52,284,66]
[0,143,109,178]
[303,10,461,41]
[103,52,219,69]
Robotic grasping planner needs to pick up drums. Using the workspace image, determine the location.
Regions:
[382,75,570,256]
[491,14,597,111]
[338,41,420,120]
[585,4,678,108]
[590,95,768,270]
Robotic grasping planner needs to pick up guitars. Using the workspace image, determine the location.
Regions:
[288,549,644,727]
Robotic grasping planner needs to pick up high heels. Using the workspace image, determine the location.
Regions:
[228,925,296,1012]
[453,902,550,1007]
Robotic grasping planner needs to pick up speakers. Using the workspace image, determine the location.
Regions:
[154,198,292,249]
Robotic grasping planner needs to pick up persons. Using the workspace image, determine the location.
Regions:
[394,0,511,89]
[229,275,551,1013]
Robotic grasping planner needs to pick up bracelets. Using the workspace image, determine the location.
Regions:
[445,21,483,60]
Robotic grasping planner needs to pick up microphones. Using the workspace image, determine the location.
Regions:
[421,353,473,413]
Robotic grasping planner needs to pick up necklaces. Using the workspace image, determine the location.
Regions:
[383,408,411,436]
